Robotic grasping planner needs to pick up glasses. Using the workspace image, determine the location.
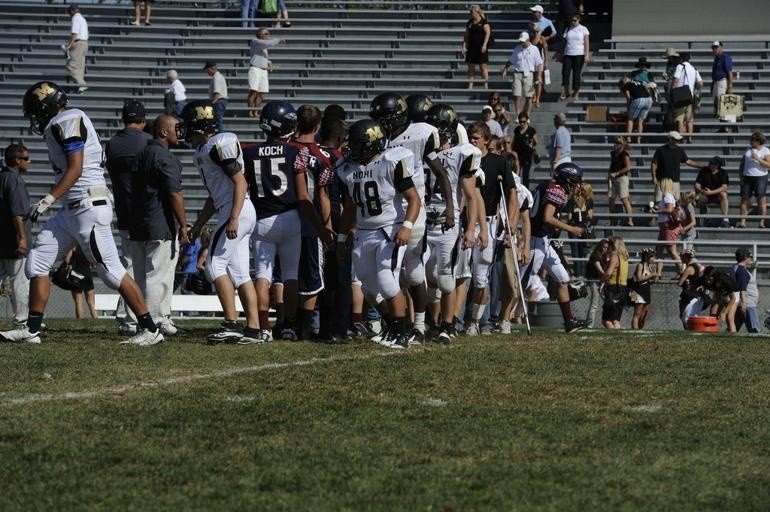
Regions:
[17,157,29,161]
[520,121,527,123]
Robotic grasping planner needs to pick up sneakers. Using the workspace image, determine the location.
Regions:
[623,223,634,226]
[130,22,141,25]
[118,317,187,347]
[514,118,519,123]
[206,320,512,348]
[80,86,87,92]
[0,326,41,344]
[721,220,766,228]
[527,119,530,123]
[566,318,593,334]
[145,22,151,25]
[16,321,46,330]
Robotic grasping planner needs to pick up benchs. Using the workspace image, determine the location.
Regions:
[0,0,770,279]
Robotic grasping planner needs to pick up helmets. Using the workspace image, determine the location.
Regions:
[348,92,458,162]
[553,163,583,194]
[260,101,298,138]
[639,248,656,254]
[679,249,695,257]
[175,100,219,138]
[201,228,213,245]
[23,81,69,135]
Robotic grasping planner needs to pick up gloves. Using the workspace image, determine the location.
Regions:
[22,193,56,223]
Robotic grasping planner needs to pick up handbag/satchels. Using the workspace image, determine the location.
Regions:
[669,206,687,226]
[693,88,700,113]
[488,32,494,47]
[534,152,541,163]
[716,94,743,120]
[555,37,566,63]
[262,0,277,14]
[670,85,693,108]
[645,82,661,102]
[543,69,552,85]
[604,285,624,305]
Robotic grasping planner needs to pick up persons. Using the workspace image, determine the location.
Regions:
[0,1,770,349]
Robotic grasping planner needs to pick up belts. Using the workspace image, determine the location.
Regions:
[67,200,106,210]
[74,39,87,42]
[617,174,627,178]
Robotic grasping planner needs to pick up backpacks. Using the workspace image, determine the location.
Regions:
[626,263,644,306]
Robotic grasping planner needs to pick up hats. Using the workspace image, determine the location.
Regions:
[634,58,652,68]
[661,48,680,59]
[66,3,78,10]
[518,32,529,42]
[530,5,544,14]
[122,101,145,120]
[554,113,566,124]
[203,61,216,70]
[712,41,723,48]
[668,131,684,140]
[482,105,493,114]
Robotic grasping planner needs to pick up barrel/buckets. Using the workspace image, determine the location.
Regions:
[686,316,721,332]
